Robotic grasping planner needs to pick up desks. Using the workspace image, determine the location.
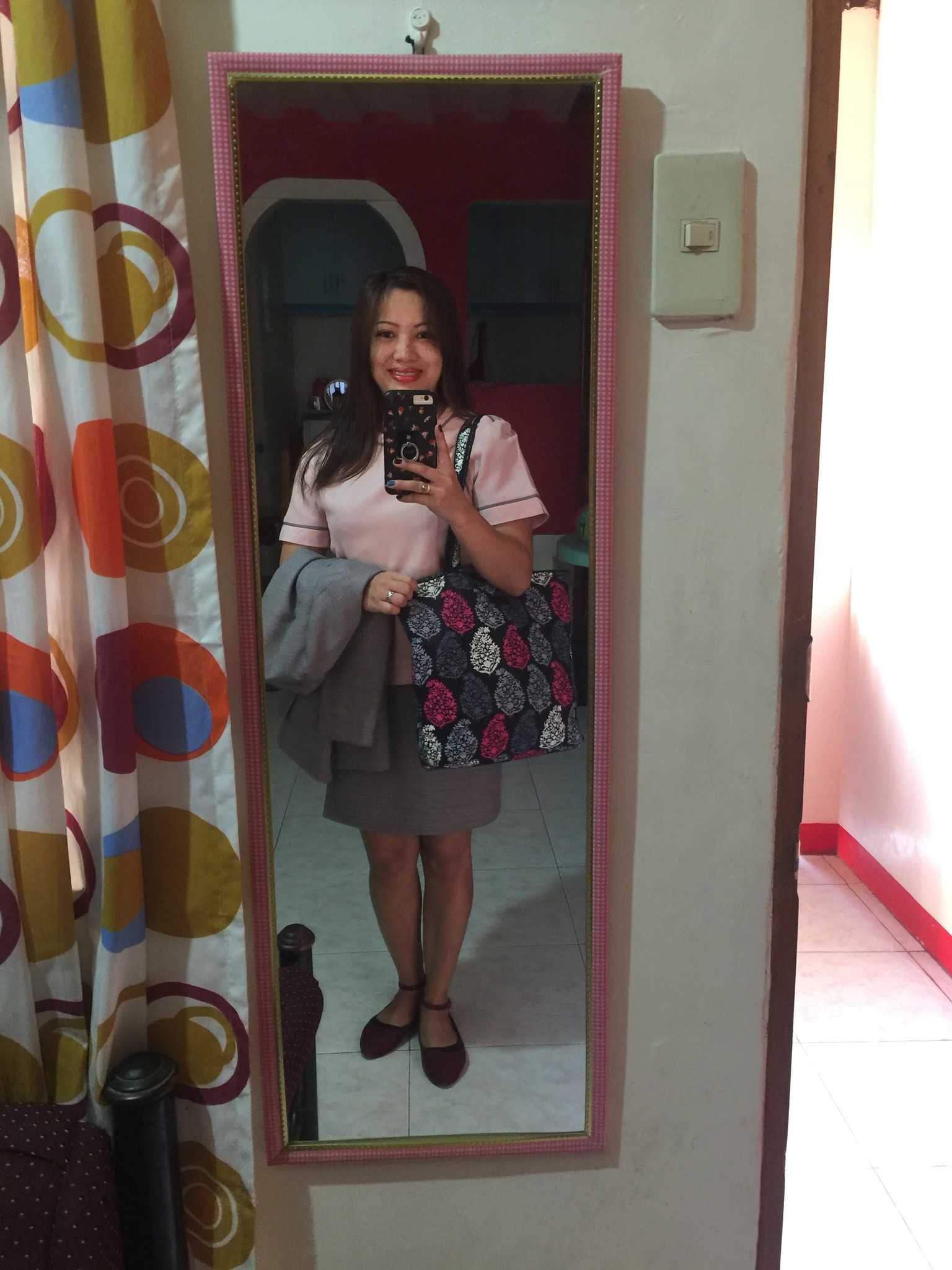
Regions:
[555,533,590,700]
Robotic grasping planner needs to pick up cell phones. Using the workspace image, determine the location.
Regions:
[383,389,438,495]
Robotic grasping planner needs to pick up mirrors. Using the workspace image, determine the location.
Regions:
[208,51,621,1166]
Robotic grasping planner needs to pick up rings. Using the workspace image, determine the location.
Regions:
[420,483,430,494]
[386,590,395,602]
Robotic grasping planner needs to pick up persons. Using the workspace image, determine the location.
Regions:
[278,265,551,1090]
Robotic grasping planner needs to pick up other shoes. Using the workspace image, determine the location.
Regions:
[360,974,423,1061]
[418,997,467,1089]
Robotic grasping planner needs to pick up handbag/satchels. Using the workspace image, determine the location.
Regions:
[399,414,582,770]
